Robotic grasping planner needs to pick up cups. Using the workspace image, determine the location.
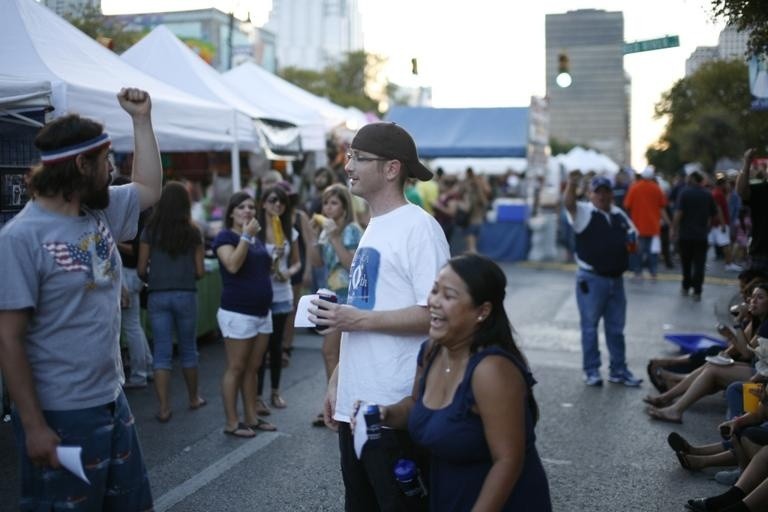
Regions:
[718,426,733,453]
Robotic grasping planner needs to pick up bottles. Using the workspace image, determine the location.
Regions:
[729,301,757,316]
[391,457,431,510]
[359,402,383,460]
[624,229,640,254]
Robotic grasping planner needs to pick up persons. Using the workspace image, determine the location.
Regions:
[117,167,370,438]
[564,148,768,511]
[0,88,163,512]
[308,122,551,511]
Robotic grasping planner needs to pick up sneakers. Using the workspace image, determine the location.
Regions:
[583,373,603,386]
[609,372,644,386]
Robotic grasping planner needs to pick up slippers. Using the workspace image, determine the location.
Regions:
[642,359,683,424]
[716,467,742,484]
[666,432,698,471]
[223,394,286,438]
[684,497,721,512]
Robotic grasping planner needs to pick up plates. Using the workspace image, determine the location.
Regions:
[705,352,733,367]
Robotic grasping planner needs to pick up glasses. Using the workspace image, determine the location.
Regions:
[346,151,389,163]
[268,195,288,205]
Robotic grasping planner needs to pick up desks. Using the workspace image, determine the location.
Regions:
[119,258,224,352]
[450,215,529,265]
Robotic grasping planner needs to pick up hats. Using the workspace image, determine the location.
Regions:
[352,124,434,181]
[590,176,612,192]
